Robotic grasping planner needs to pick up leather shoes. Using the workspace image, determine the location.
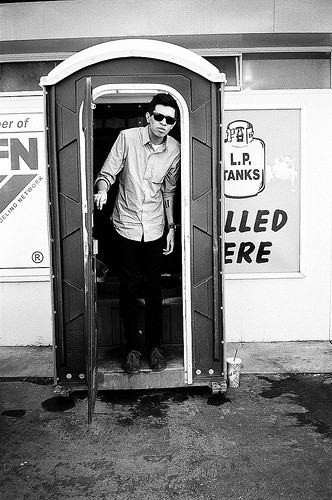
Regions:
[151,347,168,371]
[123,351,142,373]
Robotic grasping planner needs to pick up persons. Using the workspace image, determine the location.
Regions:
[92,93,181,374]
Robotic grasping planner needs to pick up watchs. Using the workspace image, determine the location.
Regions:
[169,224,177,231]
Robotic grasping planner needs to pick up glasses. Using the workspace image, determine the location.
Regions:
[151,112,177,125]
